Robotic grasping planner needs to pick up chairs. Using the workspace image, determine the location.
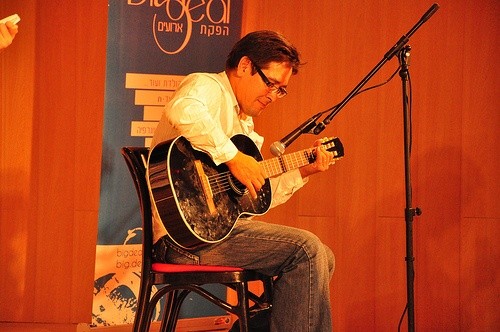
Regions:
[121,147,275,332]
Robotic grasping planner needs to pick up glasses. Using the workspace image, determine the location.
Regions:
[252,60,288,99]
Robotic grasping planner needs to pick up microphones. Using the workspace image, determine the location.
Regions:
[277,112,323,159]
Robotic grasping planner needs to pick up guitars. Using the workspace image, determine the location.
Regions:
[145,134,345,251]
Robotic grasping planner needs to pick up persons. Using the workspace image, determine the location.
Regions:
[148,31,337,332]
[0,21,18,49]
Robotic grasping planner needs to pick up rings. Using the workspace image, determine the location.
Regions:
[324,159,327,163]
